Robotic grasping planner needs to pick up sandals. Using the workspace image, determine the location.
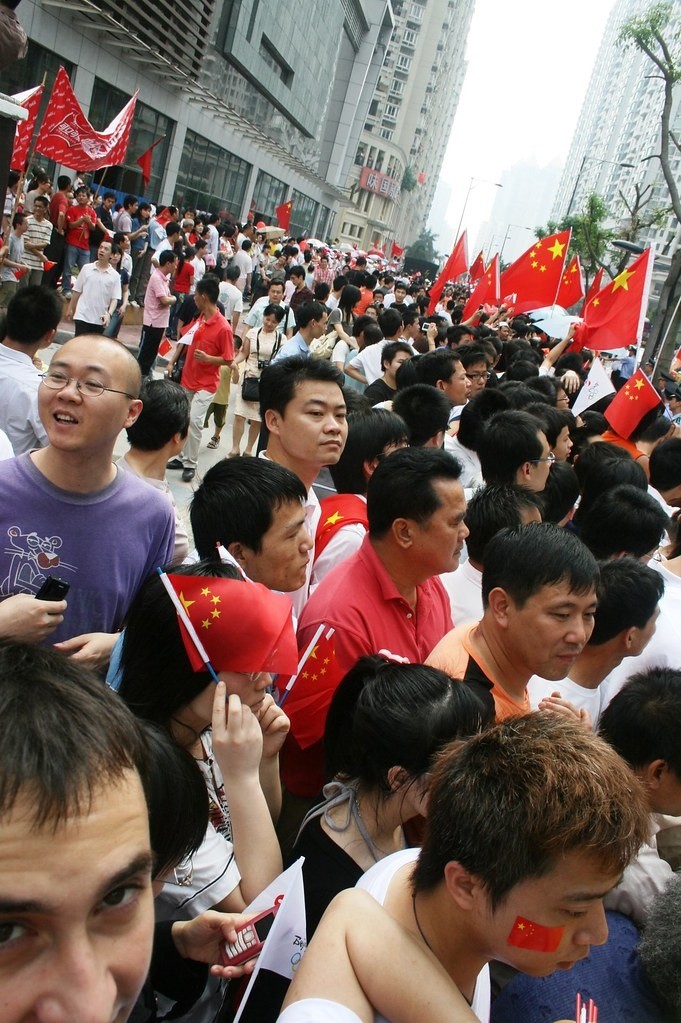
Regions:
[207,436,220,449]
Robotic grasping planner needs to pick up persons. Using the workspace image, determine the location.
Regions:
[0,165,681,1023]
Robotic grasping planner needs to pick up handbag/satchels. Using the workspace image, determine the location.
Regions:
[310,308,342,361]
[242,377,261,402]
[170,357,185,381]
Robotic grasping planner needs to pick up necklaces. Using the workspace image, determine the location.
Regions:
[413,887,472,1008]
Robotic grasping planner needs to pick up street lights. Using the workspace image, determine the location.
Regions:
[485,232,513,270]
[610,240,645,288]
[561,156,636,241]
[495,222,530,264]
[455,176,505,247]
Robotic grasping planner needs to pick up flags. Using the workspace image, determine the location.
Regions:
[568,246,651,353]
[159,339,171,356]
[163,574,297,675]
[276,201,292,234]
[45,260,56,270]
[312,495,370,565]
[462,228,603,325]
[276,627,362,749]
[428,233,467,315]
[605,368,660,440]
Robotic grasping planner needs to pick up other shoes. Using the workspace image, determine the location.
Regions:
[182,468,195,480]
[65,292,73,299]
[137,300,145,307]
[166,459,183,469]
[131,301,140,308]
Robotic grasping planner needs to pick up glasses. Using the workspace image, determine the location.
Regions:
[528,452,556,464]
[466,371,491,379]
[38,370,136,401]
[152,853,194,887]
[556,396,568,401]
[237,671,262,682]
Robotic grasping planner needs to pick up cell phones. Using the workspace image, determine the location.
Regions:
[220,904,281,966]
[34,575,70,603]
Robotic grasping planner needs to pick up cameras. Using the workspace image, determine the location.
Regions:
[422,323,430,332]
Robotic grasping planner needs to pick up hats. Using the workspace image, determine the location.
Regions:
[657,371,676,383]
[665,381,679,394]
[645,358,655,365]
[629,346,636,352]
[670,388,681,398]
